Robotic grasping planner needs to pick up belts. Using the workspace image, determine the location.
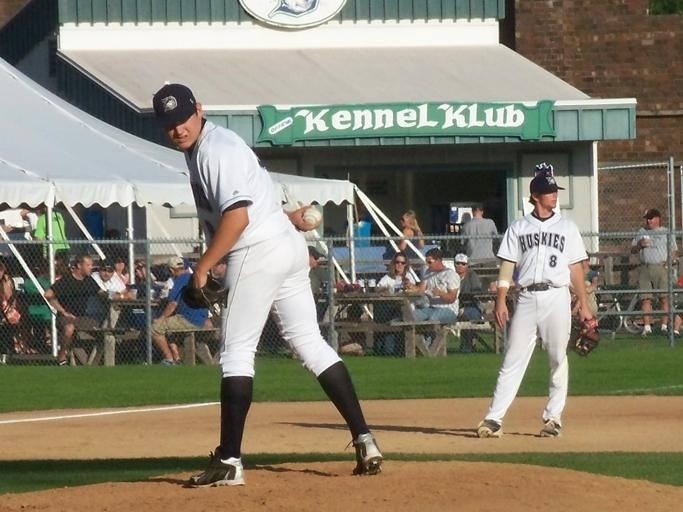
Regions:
[520,283,549,291]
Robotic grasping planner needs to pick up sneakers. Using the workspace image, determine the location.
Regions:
[352,432,383,475]
[188,446,245,489]
[540,420,561,437]
[477,421,502,438]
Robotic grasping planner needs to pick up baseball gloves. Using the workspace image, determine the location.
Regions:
[180,274,226,309]
[576,318,599,356]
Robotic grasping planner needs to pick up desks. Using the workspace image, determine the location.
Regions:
[459,290,509,355]
[100,297,198,367]
[312,290,425,359]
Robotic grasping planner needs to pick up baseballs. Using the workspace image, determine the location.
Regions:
[303,208,321,224]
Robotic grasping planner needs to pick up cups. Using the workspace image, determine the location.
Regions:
[130,289,137,300]
[401,279,409,290]
[643,235,649,247]
[320,281,327,294]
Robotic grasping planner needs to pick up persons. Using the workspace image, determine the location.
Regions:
[0,205,208,366]
[152,82,384,489]
[568,251,598,356]
[475,173,598,437]
[628,208,679,340]
[672,291,683,341]
[308,202,498,356]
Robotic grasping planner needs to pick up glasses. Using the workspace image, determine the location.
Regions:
[428,261,437,264]
[396,261,407,265]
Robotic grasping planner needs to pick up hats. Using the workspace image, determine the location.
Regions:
[308,246,325,258]
[454,253,467,264]
[167,256,184,269]
[643,209,661,218]
[102,260,115,271]
[530,174,565,193]
[153,84,196,129]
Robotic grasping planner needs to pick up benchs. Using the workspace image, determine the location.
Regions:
[0,243,655,371]
